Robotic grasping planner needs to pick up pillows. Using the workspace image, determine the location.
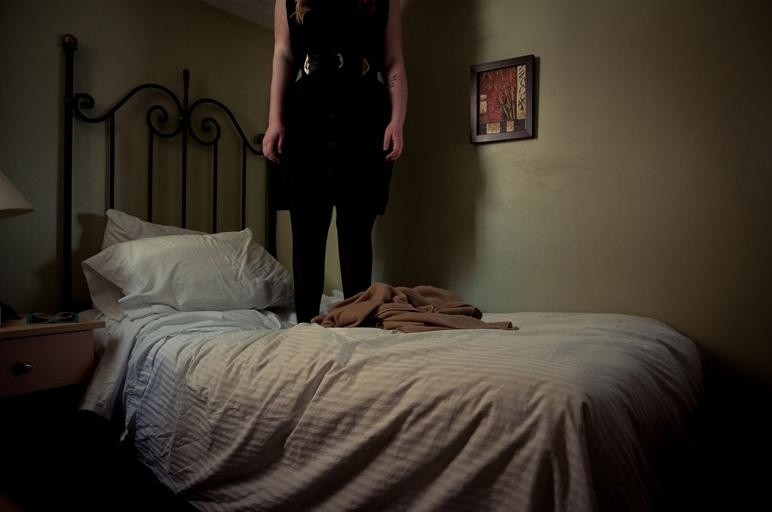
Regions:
[81,226,295,313]
[100,208,209,250]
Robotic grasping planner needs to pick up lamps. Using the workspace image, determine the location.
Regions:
[0,168,35,320]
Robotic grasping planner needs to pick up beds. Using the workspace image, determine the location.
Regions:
[55,33,707,512]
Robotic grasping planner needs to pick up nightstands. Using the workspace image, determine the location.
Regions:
[0,313,106,399]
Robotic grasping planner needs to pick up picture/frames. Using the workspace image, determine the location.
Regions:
[469,55,534,143]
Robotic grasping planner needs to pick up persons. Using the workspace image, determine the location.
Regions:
[263,1,410,327]
